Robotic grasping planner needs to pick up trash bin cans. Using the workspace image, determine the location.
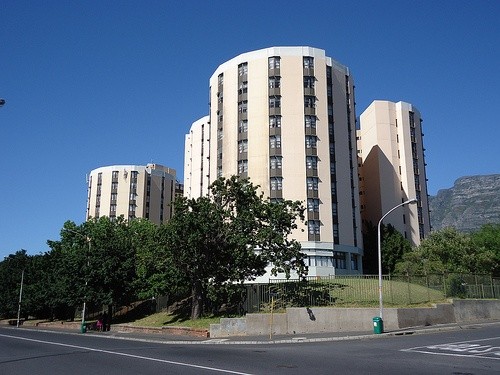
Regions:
[373,316,383,334]
[80,322,87,333]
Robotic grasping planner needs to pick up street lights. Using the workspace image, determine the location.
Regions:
[377,199,418,332]
[3,256,25,327]
[63,227,92,333]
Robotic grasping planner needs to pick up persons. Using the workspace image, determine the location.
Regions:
[96,313,104,332]
[102,311,110,331]
